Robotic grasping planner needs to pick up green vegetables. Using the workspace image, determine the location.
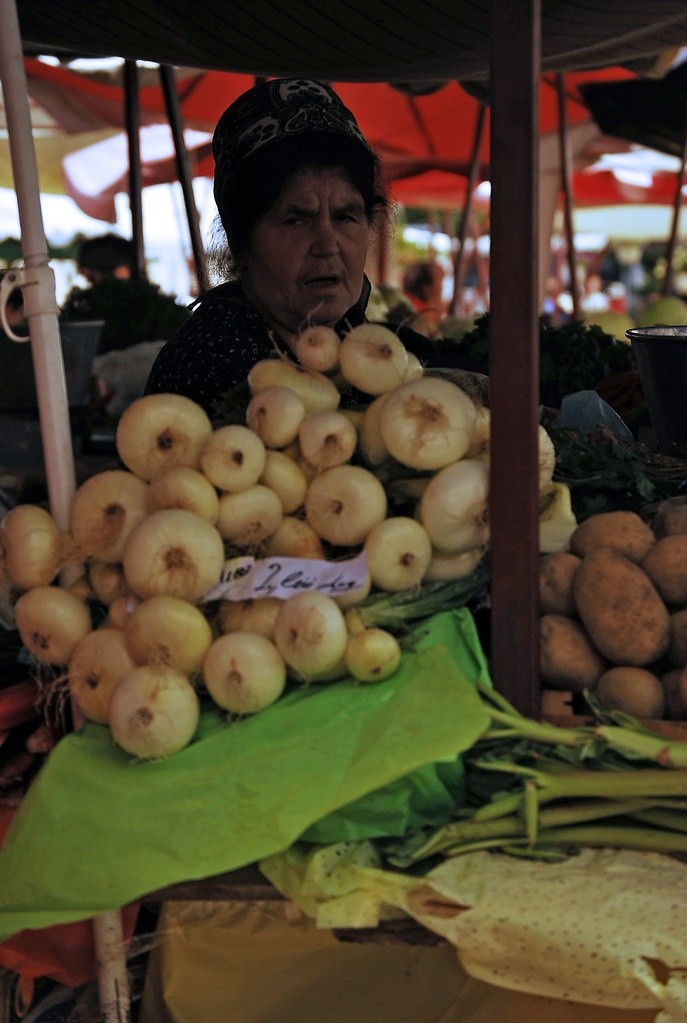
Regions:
[433,312,687,519]
[378,681,687,873]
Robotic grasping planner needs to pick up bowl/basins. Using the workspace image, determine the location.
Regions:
[0,321,104,405]
[625,325,687,460]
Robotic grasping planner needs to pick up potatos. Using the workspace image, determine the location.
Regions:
[539,496,687,726]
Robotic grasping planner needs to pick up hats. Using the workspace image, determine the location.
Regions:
[212,79,372,215]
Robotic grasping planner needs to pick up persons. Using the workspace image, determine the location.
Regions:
[134,78,434,428]
[402,258,448,321]
[0,269,86,410]
[84,289,170,422]
[56,233,188,358]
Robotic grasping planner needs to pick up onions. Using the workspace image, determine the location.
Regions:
[0,321,562,761]
